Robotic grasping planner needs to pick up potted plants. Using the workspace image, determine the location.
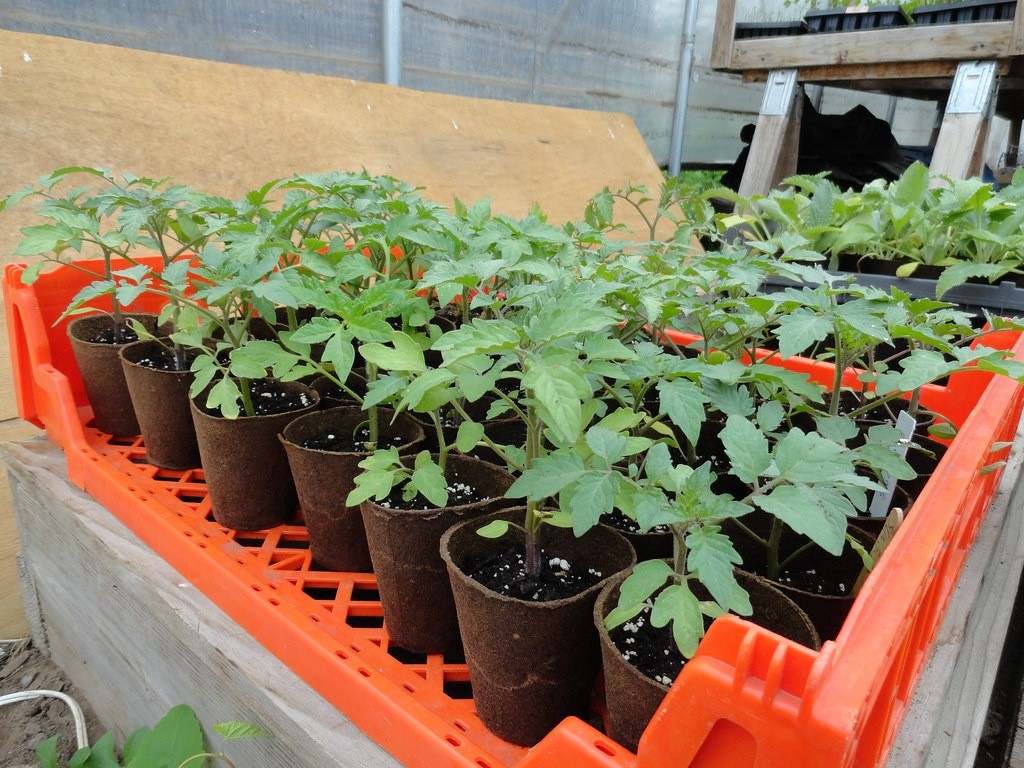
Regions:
[0,169,1024,768]
